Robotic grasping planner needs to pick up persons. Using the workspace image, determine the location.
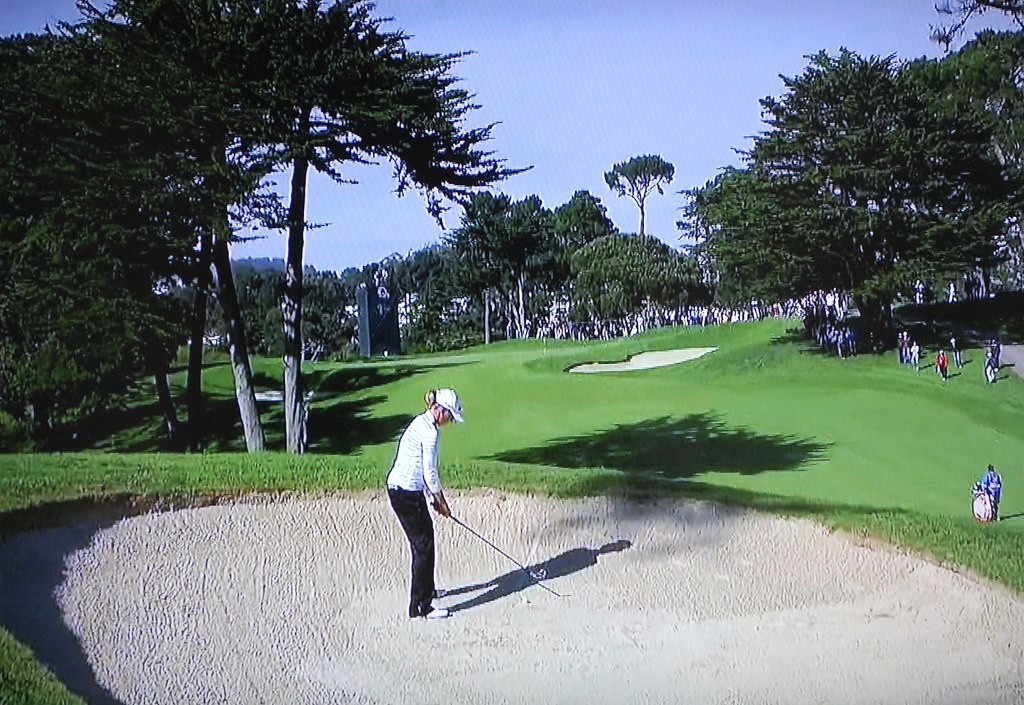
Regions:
[387,388,463,619]
[984,339,1000,385]
[541,287,856,357]
[937,349,948,380]
[949,335,963,367]
[984,463,1002,520]
[914,279,924,304]
[948,283,958,303]
[964,270,985,300]
[910,341,919,373]
[897,330,911,363]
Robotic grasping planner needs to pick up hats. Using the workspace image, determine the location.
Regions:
[431,387,469,422]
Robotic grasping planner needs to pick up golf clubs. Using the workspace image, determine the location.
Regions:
[430,500,571,597]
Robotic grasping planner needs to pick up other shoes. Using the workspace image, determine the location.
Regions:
[431,588,449,598]
[413,608,449,620]
